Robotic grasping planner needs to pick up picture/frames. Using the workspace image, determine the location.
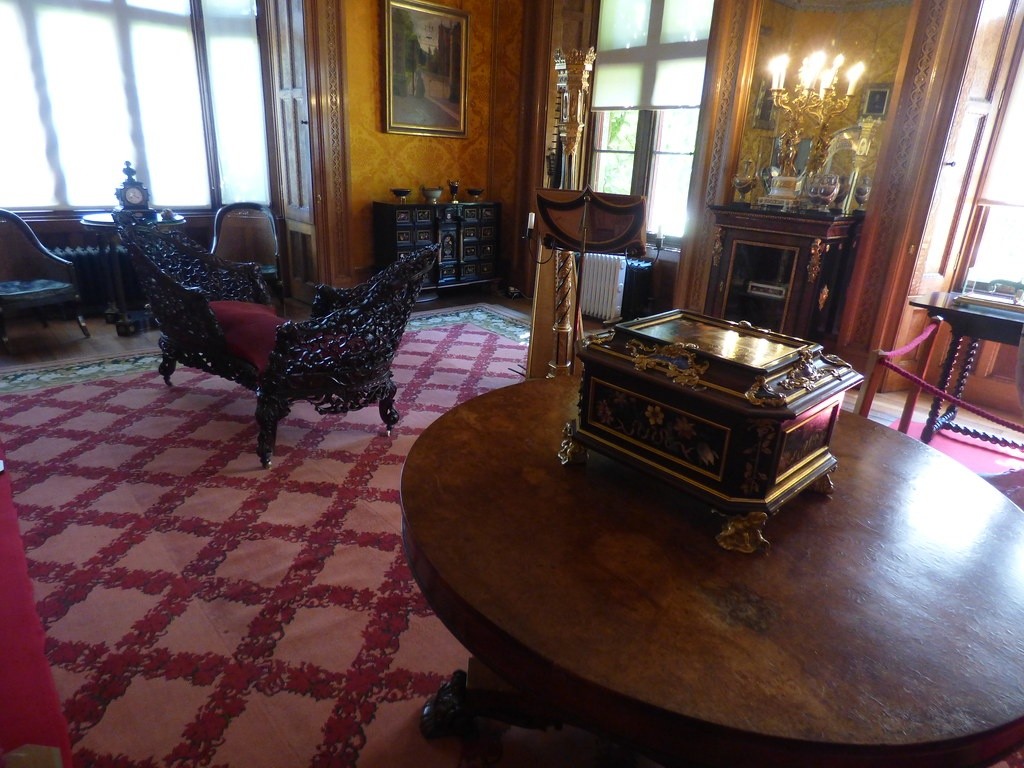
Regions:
[752,74,779,131]
[859,82,894,120]
[380,0,472,140]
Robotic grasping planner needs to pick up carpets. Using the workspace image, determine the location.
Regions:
[0,303,669,768]
[891,418,1024,475]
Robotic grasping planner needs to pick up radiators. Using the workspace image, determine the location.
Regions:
[581,252,628,329]
[620,258,653,321]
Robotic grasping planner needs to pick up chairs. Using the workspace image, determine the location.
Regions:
[0,209,92,355]
[210,202,287,318]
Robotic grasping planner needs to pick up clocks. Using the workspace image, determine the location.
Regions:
[110,160,157,225]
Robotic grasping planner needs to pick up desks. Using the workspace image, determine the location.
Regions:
[400,374,1024,768]
[80,212,187,337]
[905,290,1024,453]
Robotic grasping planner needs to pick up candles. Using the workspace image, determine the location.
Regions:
[656,222,665,237]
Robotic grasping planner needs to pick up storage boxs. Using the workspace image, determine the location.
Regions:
[556,307,866,554]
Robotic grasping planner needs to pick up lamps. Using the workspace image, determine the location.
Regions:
[797,56,866,175]
[768,49,846,173]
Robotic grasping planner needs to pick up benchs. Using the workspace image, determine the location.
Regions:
[111,212,442,473]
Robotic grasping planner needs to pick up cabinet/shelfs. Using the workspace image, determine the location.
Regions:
[372,199,505,299]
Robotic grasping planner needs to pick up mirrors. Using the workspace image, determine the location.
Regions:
[728,0,921,216]
